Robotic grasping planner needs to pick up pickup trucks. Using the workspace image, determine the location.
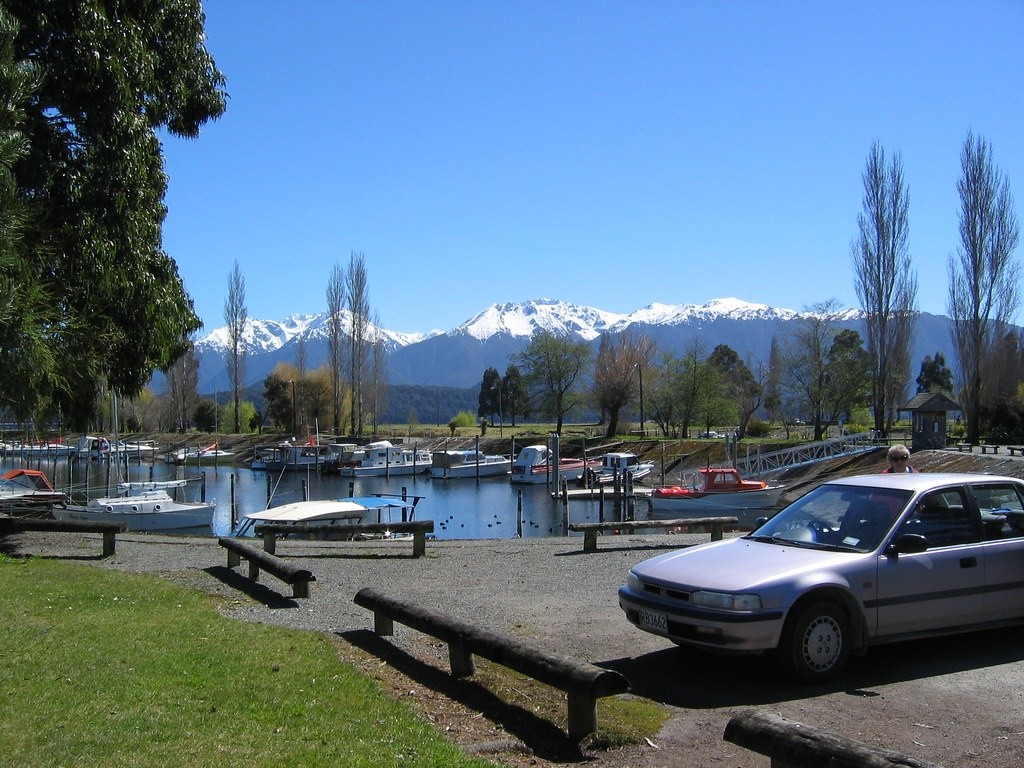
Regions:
[793,418,805,426]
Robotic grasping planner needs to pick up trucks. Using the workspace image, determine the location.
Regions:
[702,430,720,438]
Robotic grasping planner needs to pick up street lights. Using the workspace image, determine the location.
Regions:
[634,361,645,436]
[288,380,298,435]
[489,385,503,438]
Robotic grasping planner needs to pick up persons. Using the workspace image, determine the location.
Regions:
[868,443,921,518]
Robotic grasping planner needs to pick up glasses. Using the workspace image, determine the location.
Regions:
[890,454,908,462]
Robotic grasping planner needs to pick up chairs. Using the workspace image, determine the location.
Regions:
[838,500,890,547]
[926,504,1024,545]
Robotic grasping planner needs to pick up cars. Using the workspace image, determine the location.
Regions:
[618,473,1024,690]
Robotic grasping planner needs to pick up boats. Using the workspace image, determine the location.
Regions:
[250,445,336,471]
[335,447,435,477]
[648,469,786,512]
[575,451,654,484]
[0,437,236,466]
[51,388,217,532]
[508,446,604,484]
[229,496,438,539]
[429,448,517,479]
